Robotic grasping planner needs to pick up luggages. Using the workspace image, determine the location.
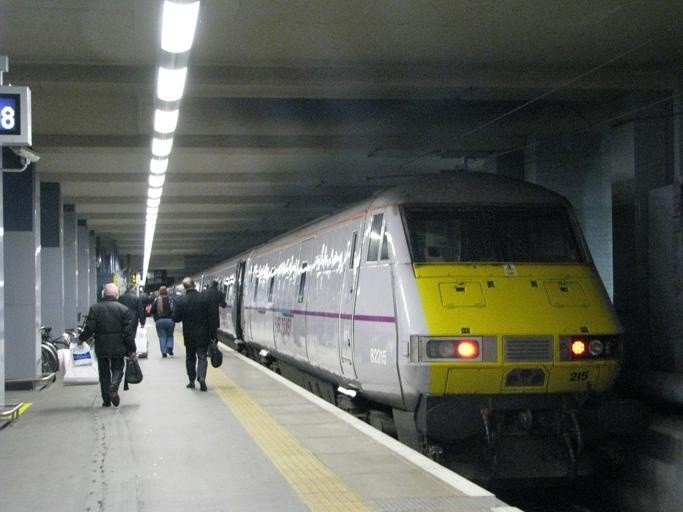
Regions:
[135,328,148,357]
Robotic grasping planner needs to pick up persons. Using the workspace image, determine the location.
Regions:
[199,279,226,357]
[117,283,144,338]
[149,286,174,358]
[75,282,137,407]
[138,286,146,328]
[170,276,218,392]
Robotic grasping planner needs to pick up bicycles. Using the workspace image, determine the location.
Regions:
[40,312,96,374]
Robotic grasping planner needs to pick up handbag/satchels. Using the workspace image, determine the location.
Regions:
[210,341,222,367]
[126,358,142,383]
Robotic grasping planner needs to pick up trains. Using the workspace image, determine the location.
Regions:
[150,171,628,499]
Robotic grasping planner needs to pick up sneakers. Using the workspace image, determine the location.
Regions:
[109,385,119,406]
[198,377,207,391]
